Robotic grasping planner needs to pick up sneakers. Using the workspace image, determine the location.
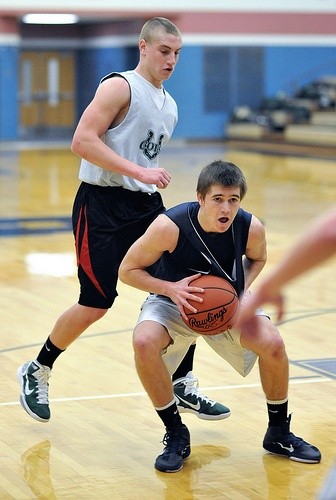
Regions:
[153,424,192,473]
[18,358,52,422]
[262,427,325,465]
[166,371,232,422]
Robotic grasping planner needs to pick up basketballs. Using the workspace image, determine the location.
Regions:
[181,275,239,336]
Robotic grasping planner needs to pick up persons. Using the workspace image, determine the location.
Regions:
[16,16,230,424]
[118,160,321,473]
[233,209,336,500]
